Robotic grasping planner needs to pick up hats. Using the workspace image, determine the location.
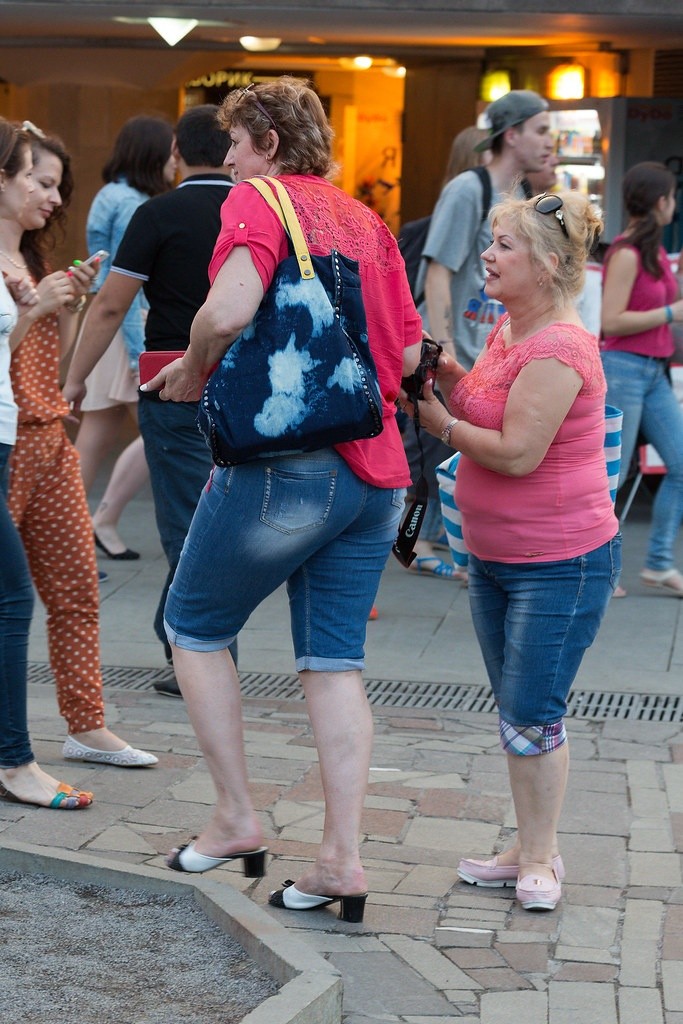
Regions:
[472,89,550,154]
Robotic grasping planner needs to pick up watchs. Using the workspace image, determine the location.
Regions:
[441,419,458,447]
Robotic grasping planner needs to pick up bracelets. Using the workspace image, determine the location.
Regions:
[665,306,673,322]
[66,295,86,313]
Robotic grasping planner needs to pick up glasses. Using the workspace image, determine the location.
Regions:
[22,120,49,142]
[526,191,573,264]
[234,82,278,133]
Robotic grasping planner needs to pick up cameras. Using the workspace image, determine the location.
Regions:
[400,340,440,400]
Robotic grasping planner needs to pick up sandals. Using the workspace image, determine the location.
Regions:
[610,585,626,598]
[637,565,683,597]
[408,552,465,580]
[429,529,452,553]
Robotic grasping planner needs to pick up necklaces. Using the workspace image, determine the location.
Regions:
[1,251,26,269]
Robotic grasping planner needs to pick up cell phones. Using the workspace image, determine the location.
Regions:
[85,250,110,264]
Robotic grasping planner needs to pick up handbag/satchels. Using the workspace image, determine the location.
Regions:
[193,174,385,468]
[428,403,624,572]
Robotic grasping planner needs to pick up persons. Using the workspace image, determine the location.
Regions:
[72,116,178,561]
[600,162,683,598]
[1,112,95,808]
[63,106,237,696]
[138,81,420,923]
[1,118,159,768]
[399,192,621,912]
[409,91,560,581]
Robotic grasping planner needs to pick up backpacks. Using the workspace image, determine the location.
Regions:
[397,167,493,312]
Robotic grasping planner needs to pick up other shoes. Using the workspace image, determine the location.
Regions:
[456,853,566,888]
[0,772,95,810]
[58,735,159,769]
[96,570,108,583]
[151,675,184,698]
[91,521,140,561]
[513,867,562,911]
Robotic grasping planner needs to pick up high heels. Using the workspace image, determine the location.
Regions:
[163,835,269,878]
[268,879,368,923]
